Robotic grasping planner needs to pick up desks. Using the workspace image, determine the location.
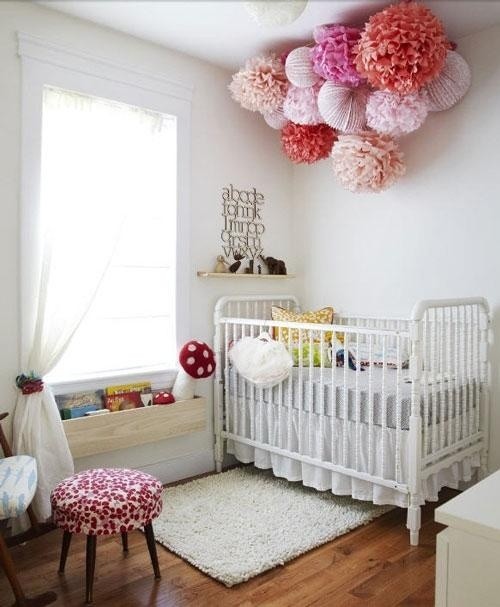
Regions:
[433,469,499,607]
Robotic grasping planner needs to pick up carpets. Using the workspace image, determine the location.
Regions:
[134,458,397,589]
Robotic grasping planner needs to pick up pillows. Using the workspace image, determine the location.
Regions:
[354,338,416,372]
[267,305,336,366]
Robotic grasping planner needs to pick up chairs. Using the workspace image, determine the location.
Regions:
[2,411,41,556]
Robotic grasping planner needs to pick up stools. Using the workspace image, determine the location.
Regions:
[49,464,165,604]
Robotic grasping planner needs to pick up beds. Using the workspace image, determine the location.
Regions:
[212,293,492,546]
